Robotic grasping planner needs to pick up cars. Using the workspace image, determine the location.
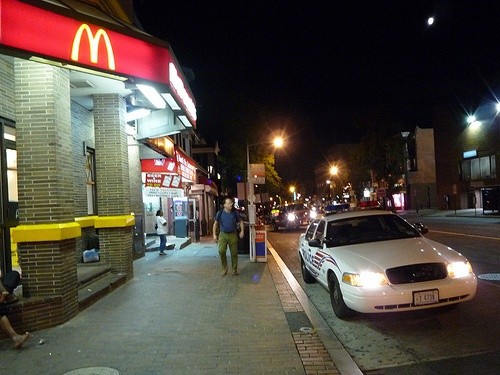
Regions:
[272,203,315,231]
[298,201,478,320]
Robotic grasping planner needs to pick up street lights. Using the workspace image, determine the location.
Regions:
[246,136,285,203]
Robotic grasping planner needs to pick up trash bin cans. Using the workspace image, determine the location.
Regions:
[173,216,189,238]
[249,224,267,263]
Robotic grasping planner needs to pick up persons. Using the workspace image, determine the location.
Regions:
[381,198,388,210]
[213,198,244,277]
[196,207,199,222]
[156,210,167,255]
[442,194,449,210]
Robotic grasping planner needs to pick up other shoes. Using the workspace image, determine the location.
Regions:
[221,271,227,276]
[159,252,167,255]
[231,270,237,276]
[14,332,29,347]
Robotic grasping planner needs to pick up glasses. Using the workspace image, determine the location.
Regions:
[226,202,233,204]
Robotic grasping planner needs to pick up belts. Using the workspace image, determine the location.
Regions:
[221,230,235,234]
